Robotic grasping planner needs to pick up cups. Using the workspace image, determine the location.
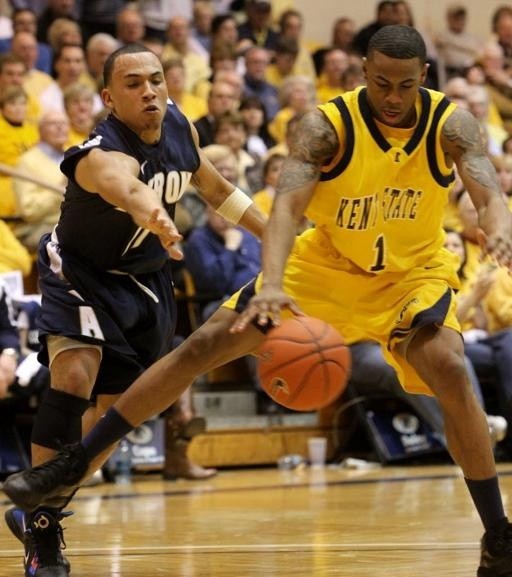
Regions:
[307,437,327,465]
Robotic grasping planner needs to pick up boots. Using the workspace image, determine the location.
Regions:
[163,408,218,482]
[172,384,205,450]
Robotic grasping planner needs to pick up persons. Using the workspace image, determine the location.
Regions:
[2,25,511,576]
[5,44,269,576]
[1,1,510,486]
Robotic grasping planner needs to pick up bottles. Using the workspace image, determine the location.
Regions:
[114,440,131,484]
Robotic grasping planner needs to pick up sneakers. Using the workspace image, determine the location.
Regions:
[3,441,93,513]
[21,497,72,577]
[3,507,24,541]
[478,516,512,577]
[485,413,508,443]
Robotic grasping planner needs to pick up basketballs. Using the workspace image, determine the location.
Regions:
[258,316,351,410]
